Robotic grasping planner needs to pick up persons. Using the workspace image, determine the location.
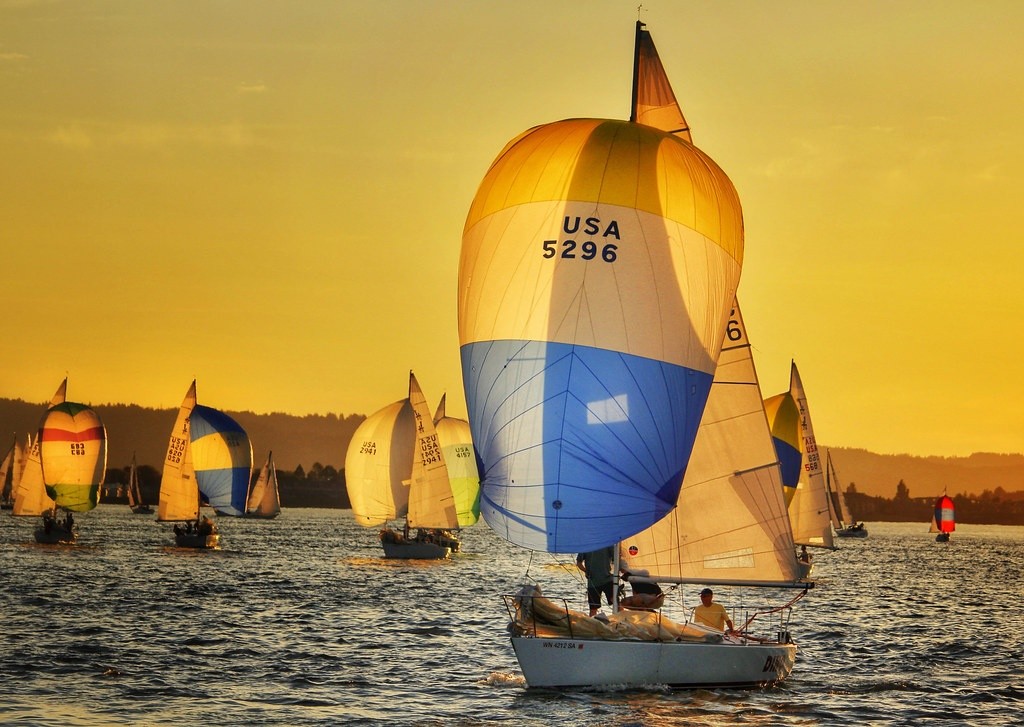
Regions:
[796,545,808,562]
[185,515,207,533]
[577,545,628,616]
[42,507,74,531]
[695,588,732,632]
[397,524,410,540]
[621,567,664,612]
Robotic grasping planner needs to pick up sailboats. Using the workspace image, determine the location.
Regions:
[345,369,482,561]
[931,494,954,547]
[161,380,222,552]
[125,454,153,515]
[4,376,109,547]
[458,4,819,688]
[824,443,870,538]
[761,357,839,585]
[188,405,281,519]
